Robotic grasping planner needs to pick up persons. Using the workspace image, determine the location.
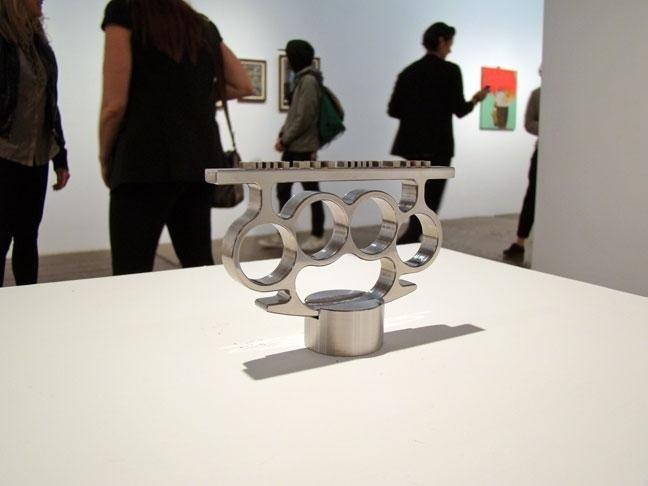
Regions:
[500,65,542,262]
[386,20,491,244]
[258,37,328,254]
[0,0,71,288]
[95,0,257,281]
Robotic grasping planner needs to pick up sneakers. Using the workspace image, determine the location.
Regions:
[300,234,326,251]
[257,233,283,249]
[504,243,524,254]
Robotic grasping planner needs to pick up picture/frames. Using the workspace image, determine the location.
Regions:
[237,54,321,112]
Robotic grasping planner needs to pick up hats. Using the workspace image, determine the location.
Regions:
[278,40,313,51]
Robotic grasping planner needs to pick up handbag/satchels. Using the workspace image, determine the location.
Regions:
[212,151,244,206]
[319,88,345,146]
